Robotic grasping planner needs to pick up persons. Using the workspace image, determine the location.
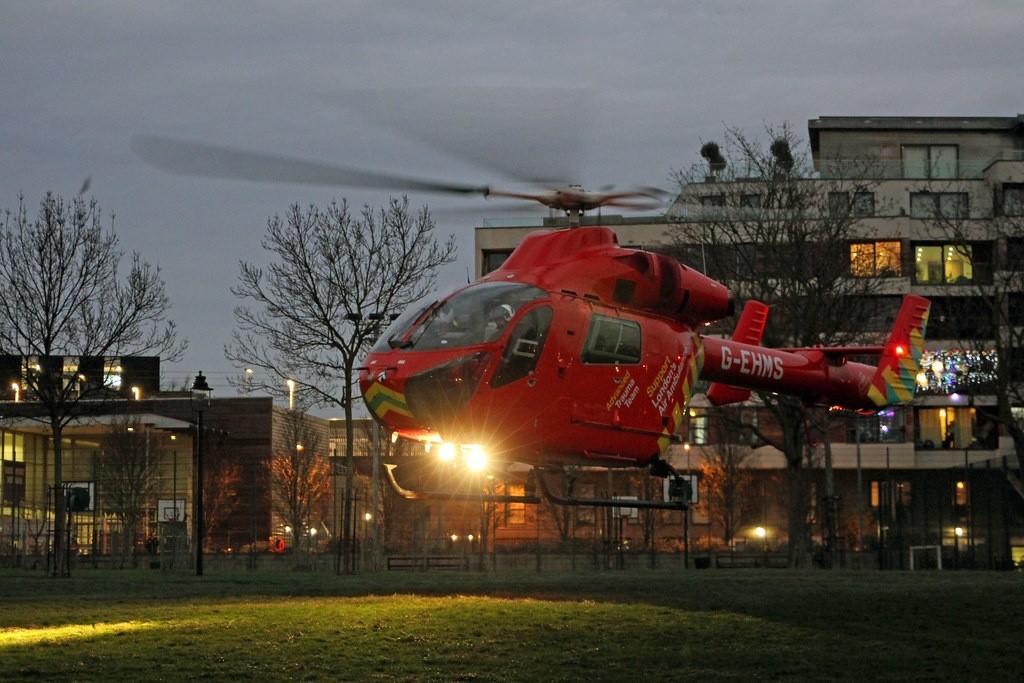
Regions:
[146,531,160,570]
[470,304,537,376]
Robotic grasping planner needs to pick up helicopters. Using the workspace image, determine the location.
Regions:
[128,91,931,509]
